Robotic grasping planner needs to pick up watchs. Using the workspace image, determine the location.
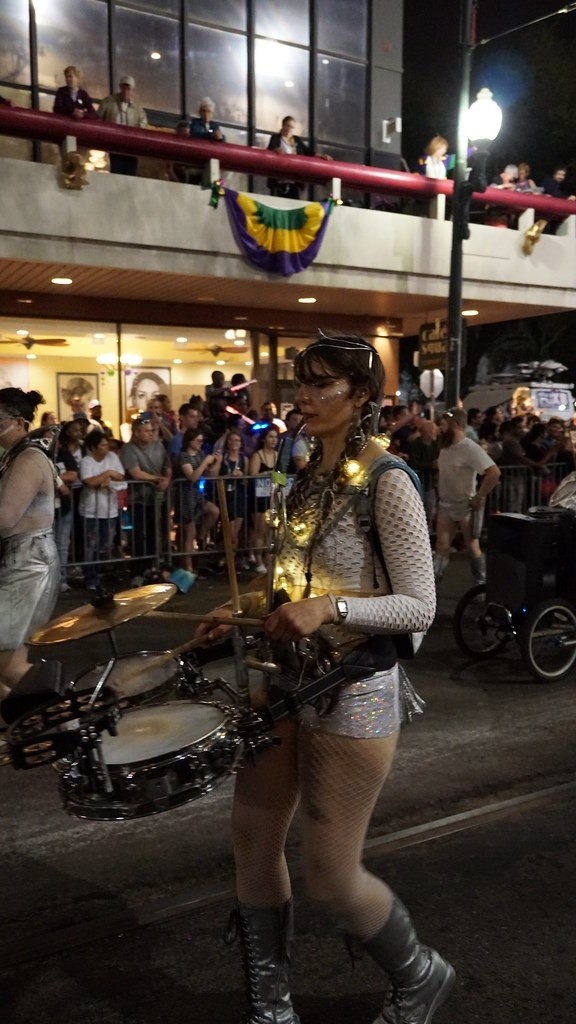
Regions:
[336,596,348,625]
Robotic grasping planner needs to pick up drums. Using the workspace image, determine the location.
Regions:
[67,649,183,703]
[53,700,244,822]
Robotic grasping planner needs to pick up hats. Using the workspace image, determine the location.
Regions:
[443,406,468,427]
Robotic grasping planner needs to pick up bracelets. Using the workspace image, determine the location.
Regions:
[477,494,485,499]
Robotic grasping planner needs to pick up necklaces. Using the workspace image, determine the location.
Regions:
[360,443,367,454]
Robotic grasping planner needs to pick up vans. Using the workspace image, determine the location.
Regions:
[462,361,576,425]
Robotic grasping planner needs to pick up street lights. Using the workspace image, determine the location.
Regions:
[445,86,503,414]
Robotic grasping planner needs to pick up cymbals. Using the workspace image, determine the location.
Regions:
[0,686,124,770]
[24,583,179,644]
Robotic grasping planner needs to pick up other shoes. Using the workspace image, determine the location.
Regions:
[56,541,275,599]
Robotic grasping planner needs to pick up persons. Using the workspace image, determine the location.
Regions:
[379,386,576,595]
[97,76,148,175]
[131,372,164,410]
[267,117,333,199]
[413,135,477,216]
[37,371,312,594]
[62,379,93,413]
[0,388,82,732]
[52,66,99,156]
[165,98,224,185]
[194,335,457,1023]
[484,163,551,229]
[542,167,576,201]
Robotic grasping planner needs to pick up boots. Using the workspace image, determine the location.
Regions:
[237,896,302,1024]
[359,892,455,1024]
